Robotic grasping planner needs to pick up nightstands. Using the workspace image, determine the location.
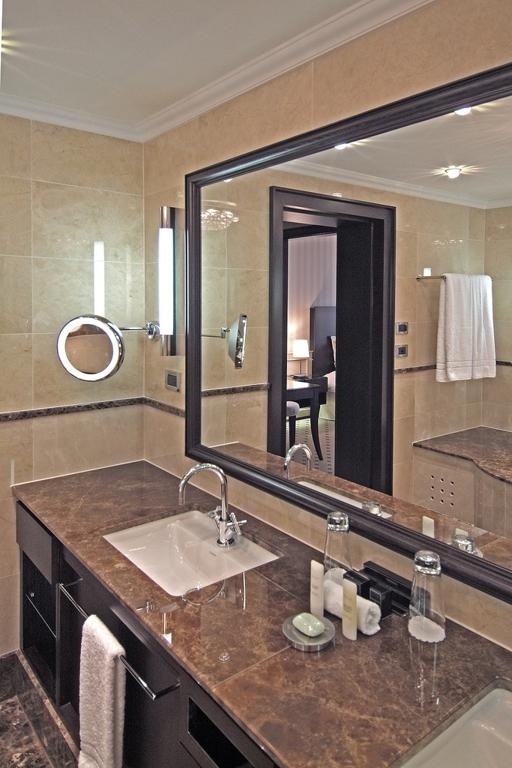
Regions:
[292,374,328,408]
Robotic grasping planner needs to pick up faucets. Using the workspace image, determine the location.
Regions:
[282,444,322,477]
[177,462,248,546]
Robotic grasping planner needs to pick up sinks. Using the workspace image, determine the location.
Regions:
[291,479,392,522]
[102,511,281,597]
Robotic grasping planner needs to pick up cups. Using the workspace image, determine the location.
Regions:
[323,513,352,575]
[410,550,446,643]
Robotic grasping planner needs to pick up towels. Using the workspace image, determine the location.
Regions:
[434,272,498,384]
[318,572,381,636]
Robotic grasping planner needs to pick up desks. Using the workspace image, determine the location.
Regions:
[285,377,323,462]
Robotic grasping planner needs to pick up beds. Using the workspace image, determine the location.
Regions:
[308,305,336,423]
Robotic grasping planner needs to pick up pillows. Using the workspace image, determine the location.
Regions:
[330,334,336,369]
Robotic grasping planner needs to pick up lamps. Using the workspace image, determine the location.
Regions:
[201,313,251,369]
[200,200,239,231]
[55,311,158,384]
[291,338,311,383]
[445,168,461,180]
[155,201,179,359]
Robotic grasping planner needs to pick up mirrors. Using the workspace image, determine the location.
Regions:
[185,60,512,608]
